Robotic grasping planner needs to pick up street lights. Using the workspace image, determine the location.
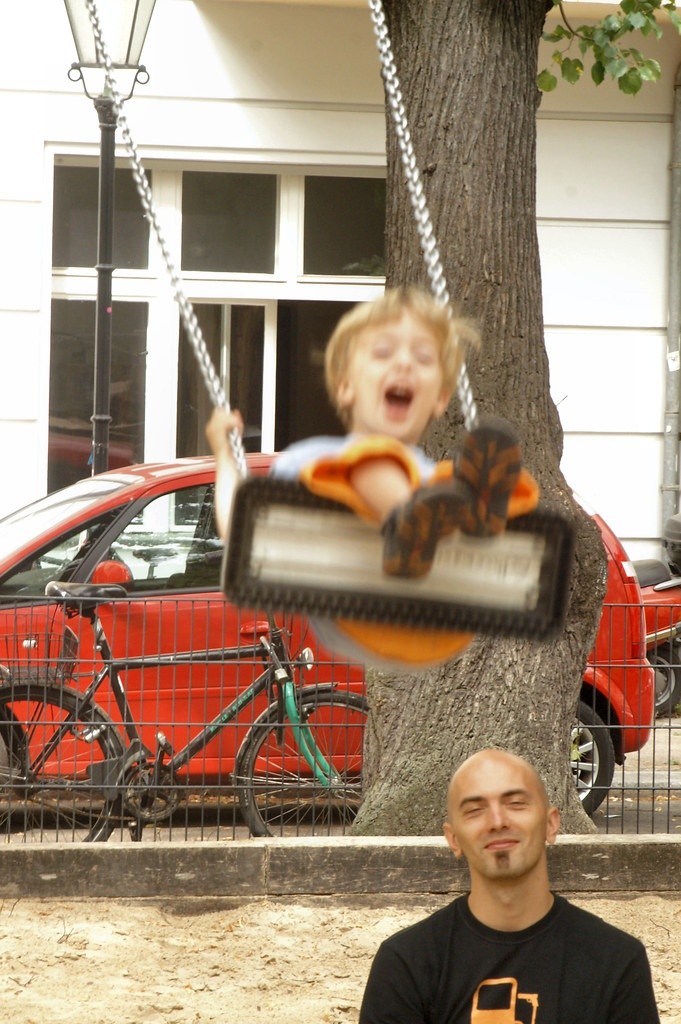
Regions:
[64,0,158,472]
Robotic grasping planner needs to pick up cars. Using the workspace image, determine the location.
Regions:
[2,447,655,812]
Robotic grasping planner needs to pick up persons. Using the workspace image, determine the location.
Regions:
[206,289,538,669]
[360,747,663,1024]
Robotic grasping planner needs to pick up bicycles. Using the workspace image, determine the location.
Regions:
[1,580,370,842]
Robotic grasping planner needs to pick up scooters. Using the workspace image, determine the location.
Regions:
[629,559,680,716]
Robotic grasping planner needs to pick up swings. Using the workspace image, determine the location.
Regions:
[89,1,575,643]
